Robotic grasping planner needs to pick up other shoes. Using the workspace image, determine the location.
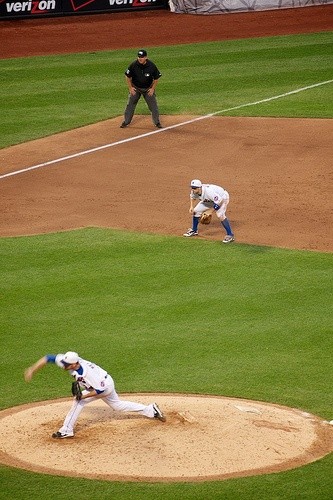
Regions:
[156,122,161,128]
[222,234,235,243]
[120,122,126,128]
[183,228,198,236]
[52,431,75,438]
[152,403,166,422]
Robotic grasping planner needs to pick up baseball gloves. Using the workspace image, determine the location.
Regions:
[200,213,212,225]
[72,380,82,401]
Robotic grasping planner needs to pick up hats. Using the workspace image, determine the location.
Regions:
[138,50,147,57]
[60,351,80,364]
[190,180,202,188]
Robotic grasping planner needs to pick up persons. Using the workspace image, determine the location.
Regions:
[23,351,166,439]
[183,178,235,243]
[120,49,162,128]
